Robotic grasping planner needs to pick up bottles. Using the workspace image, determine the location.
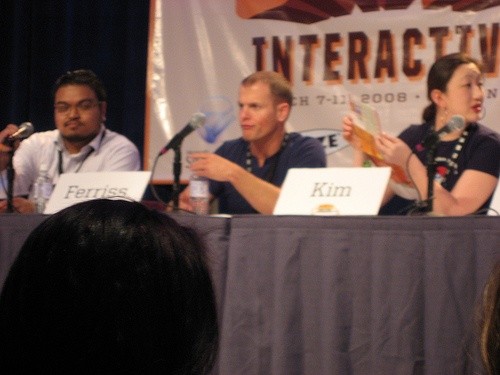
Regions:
[33,166,55,215]
[191,171,209,214]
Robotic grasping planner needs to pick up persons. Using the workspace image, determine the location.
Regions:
[0,70,141,215]
[341,53,500,217]
[167,70,326,215]
[0,199,218,375]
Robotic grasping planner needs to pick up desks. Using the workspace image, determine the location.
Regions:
[0,211,500,374]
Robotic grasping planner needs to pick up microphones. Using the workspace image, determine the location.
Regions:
[418,116,464,149]
[3,122,33,144]
[158,113,206,156]
